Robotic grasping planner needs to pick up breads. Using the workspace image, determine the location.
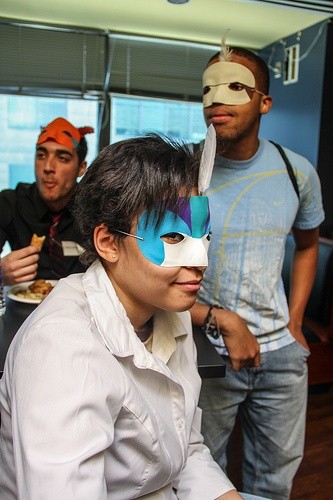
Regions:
[30,233,46,248]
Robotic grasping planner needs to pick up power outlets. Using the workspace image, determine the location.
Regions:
[274,61,281,79]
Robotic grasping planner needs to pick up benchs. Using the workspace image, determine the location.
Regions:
[282,232,333,394]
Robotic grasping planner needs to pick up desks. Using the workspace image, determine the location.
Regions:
[0,285,226,378]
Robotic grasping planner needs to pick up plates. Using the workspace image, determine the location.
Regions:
[7,280,59,304]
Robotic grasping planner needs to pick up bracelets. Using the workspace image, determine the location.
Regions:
[202,304,223,340]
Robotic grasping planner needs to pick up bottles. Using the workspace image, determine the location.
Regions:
[0,257,6,316]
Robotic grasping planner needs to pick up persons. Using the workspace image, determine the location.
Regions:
[181,45,324,500]
[0,131,272,500]
[0,117,95,379]
